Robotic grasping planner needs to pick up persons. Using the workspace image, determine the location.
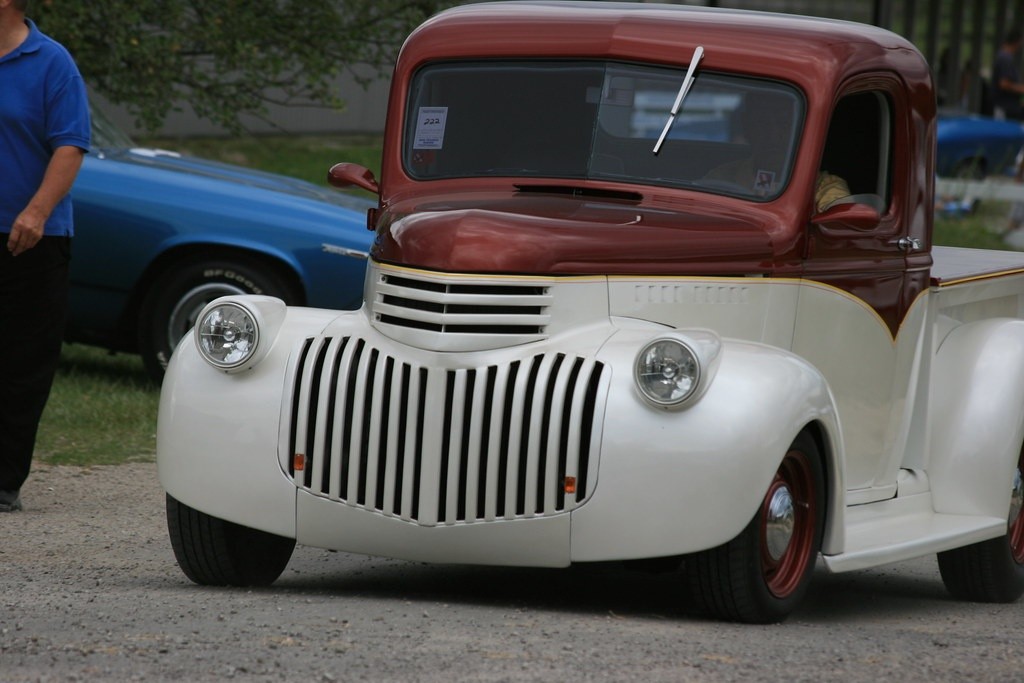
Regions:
[691,82,854,214]
[0,1,85,513]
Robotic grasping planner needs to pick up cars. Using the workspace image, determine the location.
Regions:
[156,0,1024,623]
[62,102,380,386]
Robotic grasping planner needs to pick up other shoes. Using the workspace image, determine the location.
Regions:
[0,488,21,512]
